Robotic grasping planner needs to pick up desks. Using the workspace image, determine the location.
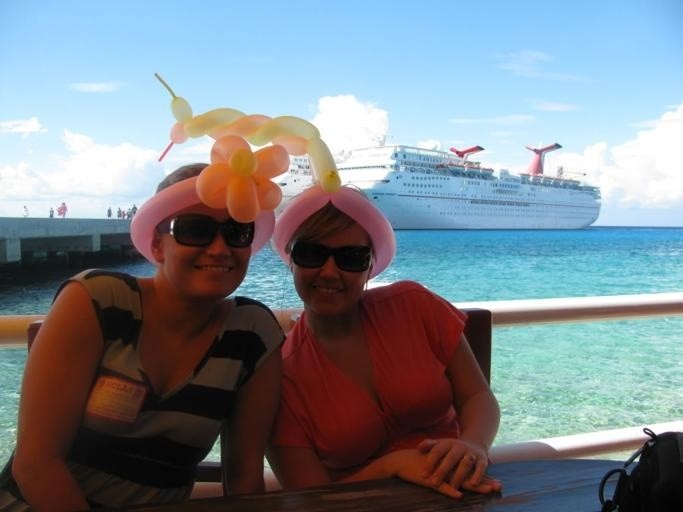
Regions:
[140,459,644,512]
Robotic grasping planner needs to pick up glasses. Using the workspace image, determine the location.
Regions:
[290,239,372,272]
[157,213,255,247]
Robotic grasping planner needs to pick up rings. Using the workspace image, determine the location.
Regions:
[463,453,476,464]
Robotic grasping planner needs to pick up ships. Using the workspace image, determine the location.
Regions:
[267,140,602,230]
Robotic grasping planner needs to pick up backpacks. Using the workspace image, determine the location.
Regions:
[599,428,683,512]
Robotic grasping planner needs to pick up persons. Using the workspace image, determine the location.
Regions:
[48,202,68,218]
[259,184,506,497]
[0,162,283,511]
[106,201,138,222]
[22,206,28,218]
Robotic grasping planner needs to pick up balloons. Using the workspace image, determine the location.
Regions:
[154,66,396,285]
[127,136,291,269]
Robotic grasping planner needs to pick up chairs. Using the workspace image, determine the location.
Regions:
[27,319,232,497]
[457,307,492,388]
